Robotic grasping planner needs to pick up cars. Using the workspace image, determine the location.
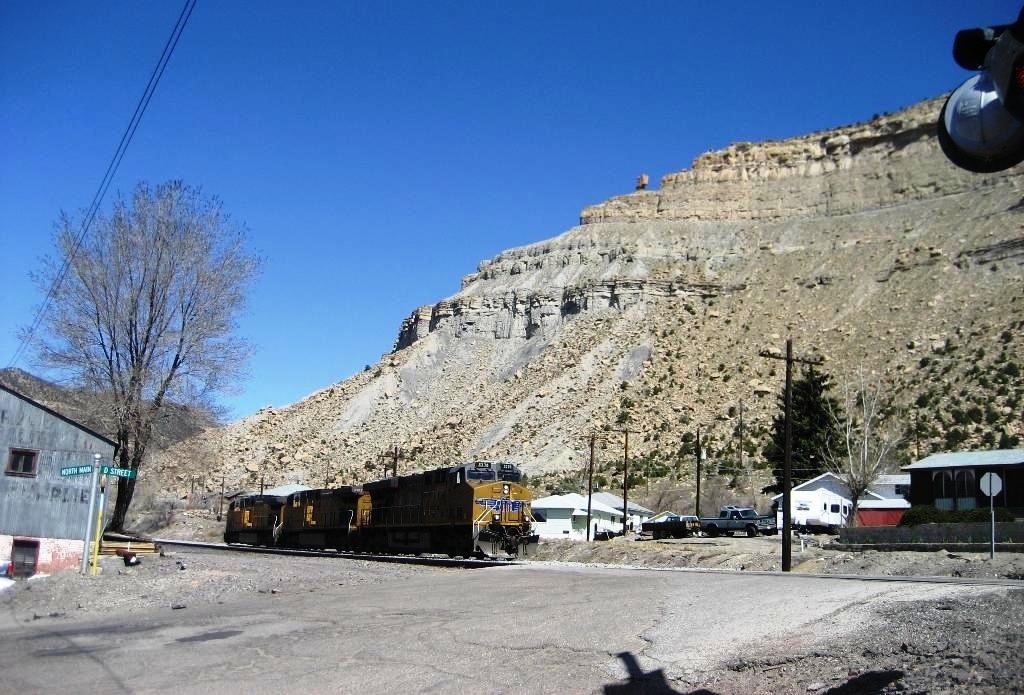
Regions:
[0,561,13,579]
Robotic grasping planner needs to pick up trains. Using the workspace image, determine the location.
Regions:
[224,460,540,562]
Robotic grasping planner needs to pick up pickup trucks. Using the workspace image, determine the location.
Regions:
[700,506,777,538]
[641,516,700,540]
